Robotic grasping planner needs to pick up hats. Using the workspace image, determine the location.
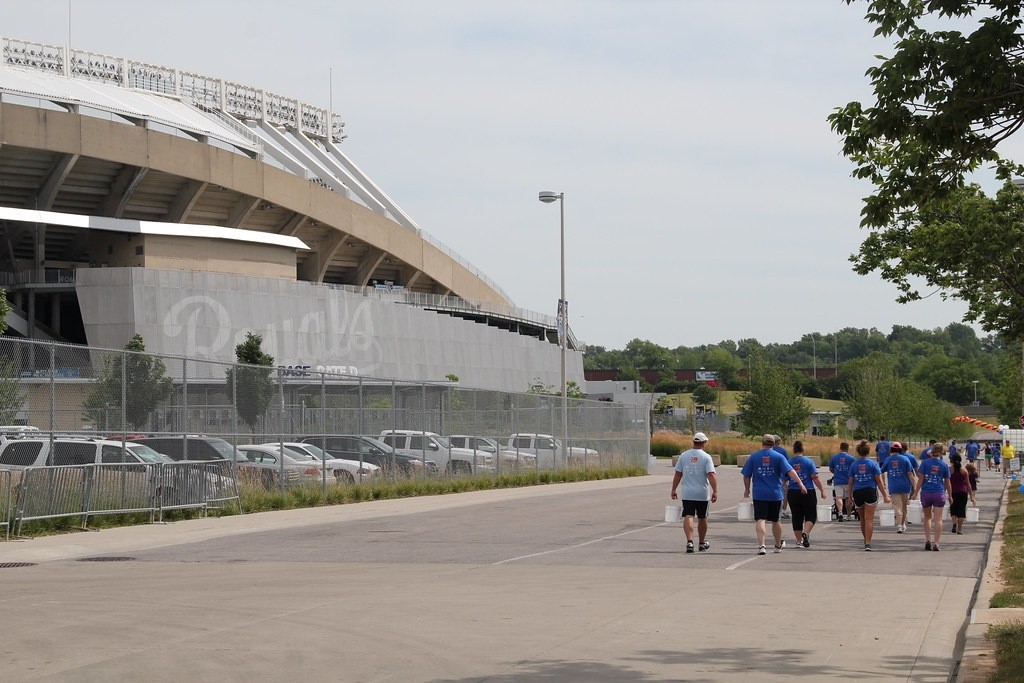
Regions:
[694,433,708,442]
[763,434,782,444]
[793,441,802,447]
[891,442,902,449]
[927,450,932,457]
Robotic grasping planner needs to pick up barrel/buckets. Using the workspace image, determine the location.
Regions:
[906,499,923,524]
[877,501,895,527]
[737,496,754,521]
[942,504,952,521]
[815,497,832,521]
[665,497,684,523]
[965,508,979,523]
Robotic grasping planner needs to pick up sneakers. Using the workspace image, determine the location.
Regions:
[699,542,709,550]
[686,542,694,553]
[774,540,786,553]
[795,540,804,547]
[758,545,767,554]
[897,524,907,533]
[801,532,810,547]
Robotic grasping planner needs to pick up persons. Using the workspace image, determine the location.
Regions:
[919,439,943,464]
[782,440,826,548]
[847,439,890,551]
[900,442,920,496]
[978,441,1001,472]
[829,442,855,521]
[910,442,953,551]
[965,464,980,500]
[948,453,976,534]
[964,439,978,464]
[949,441,956,463]
[881,441,916,533]
[772,434,790,519]
[671,432,718,553]
[875,436,890,489]
[741,434,807,555]
[1001,440,1016,479]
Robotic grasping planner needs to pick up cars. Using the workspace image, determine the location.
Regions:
[235,444,337,485]
[264,442,383,485]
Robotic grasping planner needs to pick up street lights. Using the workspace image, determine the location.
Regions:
[539,191,568,472]
[972,381,979,402]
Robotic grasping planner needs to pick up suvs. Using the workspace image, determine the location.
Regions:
[111,438,301,489]
[508,433,599,471]
[378,429,496,479]
[449,435,539,471]
[0,431,227,509]
[294,435,440,479]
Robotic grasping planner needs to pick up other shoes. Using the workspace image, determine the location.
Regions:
[933,543,940,551]
[865,544,870,551]
[924,540,933,550]
[845,516,851,521]
[781,511,790,519]
[957,530,963,534]
[838,511,844,522]
[951,523,957,533]
[998,468,1000,472]
[1003,474,1006,479]
